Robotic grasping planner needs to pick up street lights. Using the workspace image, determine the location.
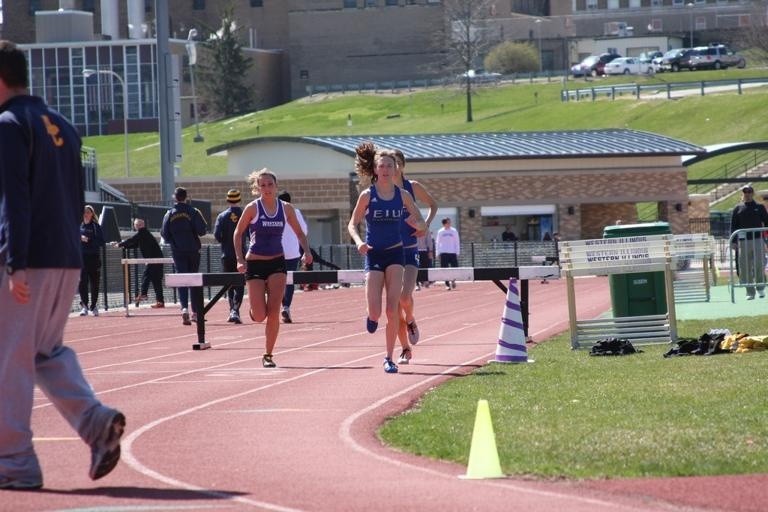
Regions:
[185,27,203,142]
[82,67,136,223]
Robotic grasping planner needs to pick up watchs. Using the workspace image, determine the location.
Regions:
[6,266,26,275]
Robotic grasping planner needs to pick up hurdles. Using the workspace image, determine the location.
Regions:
[163,266,561,349]
[531,254,558,281]
[558,233,715,352]
[121,258,176,317]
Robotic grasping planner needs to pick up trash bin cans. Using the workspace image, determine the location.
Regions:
[603,221,672,346]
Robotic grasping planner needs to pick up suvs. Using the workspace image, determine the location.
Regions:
[653,48,691,73]
[690,44,745,70]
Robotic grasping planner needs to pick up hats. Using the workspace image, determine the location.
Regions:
[226,188,241,203]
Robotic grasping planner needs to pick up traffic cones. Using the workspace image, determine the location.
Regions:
[460,399,506,481]
[487,277,531,364]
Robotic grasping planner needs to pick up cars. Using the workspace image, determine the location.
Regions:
[570,53,621,77]
[604,57,652,75]
[459,68,501,82]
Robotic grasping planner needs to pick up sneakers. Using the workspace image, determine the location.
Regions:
[1,475,43,490]
[88,413,125,481]
[367,319,419,373]
[80,295,292,367]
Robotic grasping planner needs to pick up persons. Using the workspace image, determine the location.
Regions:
[115,218,166,309]
[160,188,209,325]
[410,215,563,292]
[233,170,309,374]
[347,142,428,372]
[78,206,103,317]
[215,188,250,324]
[756,190,768,284]
[730,186,768,301]
[1,41,128,490]
[734,248,747,289]
[277,189,307,323]
[389,148,439,364]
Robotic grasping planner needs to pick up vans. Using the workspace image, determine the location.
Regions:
[639,51,662,61]
